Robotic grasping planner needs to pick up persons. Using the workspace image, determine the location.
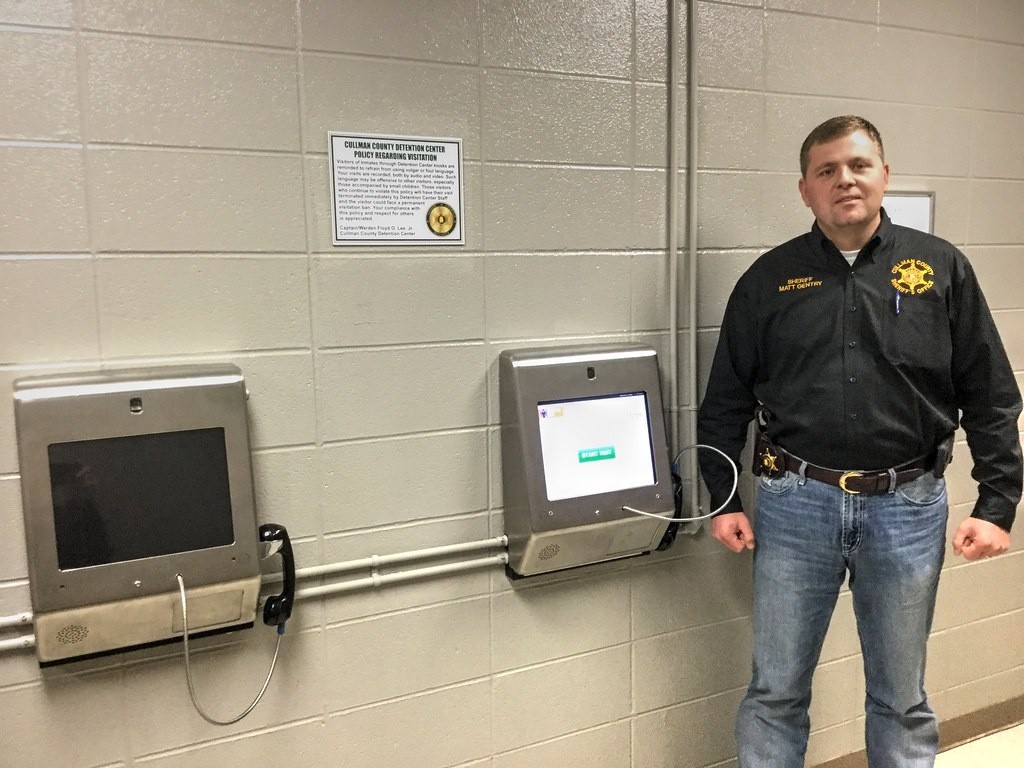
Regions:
[696,114,1024,768]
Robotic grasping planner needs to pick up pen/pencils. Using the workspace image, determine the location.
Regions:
[895,289,901,314]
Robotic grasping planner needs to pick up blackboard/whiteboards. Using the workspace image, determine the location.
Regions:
[879,190,937,237]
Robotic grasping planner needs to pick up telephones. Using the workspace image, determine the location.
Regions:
[499,340,684,582]
[13,360,296,670]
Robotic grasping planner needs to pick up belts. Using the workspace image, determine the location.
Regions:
[781,449,940,496]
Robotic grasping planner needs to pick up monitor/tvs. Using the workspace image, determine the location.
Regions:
[47,427,235,570]
[537,391,657,501]
